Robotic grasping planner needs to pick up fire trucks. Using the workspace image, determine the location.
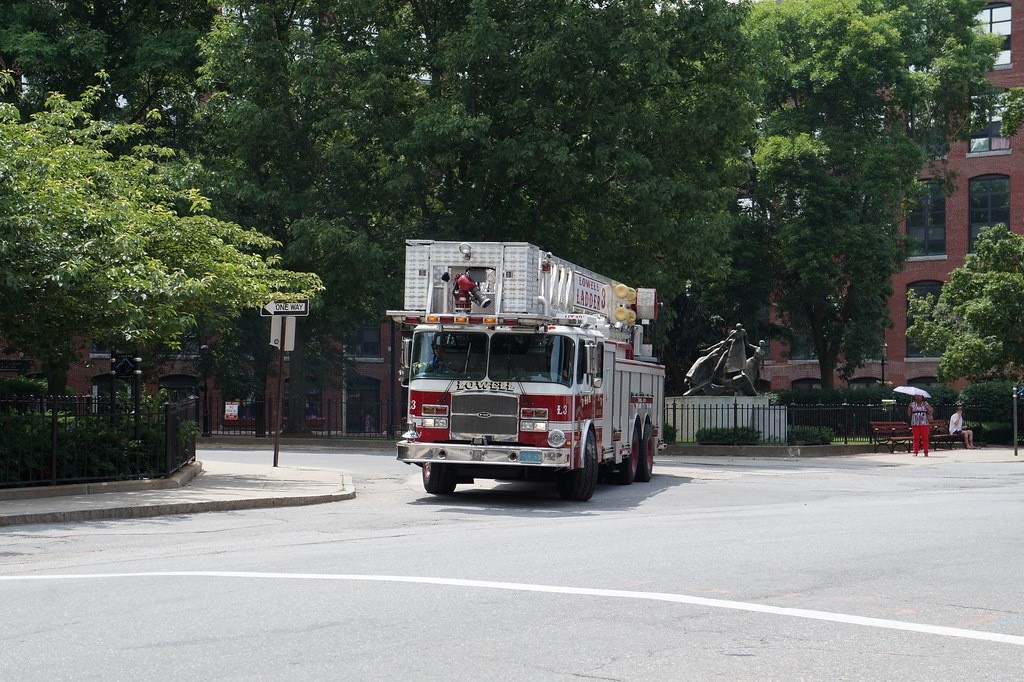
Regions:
[387,238,666,501]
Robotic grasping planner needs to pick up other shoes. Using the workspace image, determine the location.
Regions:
[967,446,975,449]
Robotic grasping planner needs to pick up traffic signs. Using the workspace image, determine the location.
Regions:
[260,299,310,318]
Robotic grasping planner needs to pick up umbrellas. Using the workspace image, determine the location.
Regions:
[893,386,931,402]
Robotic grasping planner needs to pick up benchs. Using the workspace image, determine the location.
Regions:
[869,419,967,454]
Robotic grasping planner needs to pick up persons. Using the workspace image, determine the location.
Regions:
[425,345,449,373]
[949,407,978,448]
[908,395,934,457]
[684,323,765,388]
[538,343,571,378]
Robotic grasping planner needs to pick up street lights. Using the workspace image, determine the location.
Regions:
[878,341,888,386]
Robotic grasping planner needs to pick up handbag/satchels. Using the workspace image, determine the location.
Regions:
[928,415,933,423]
